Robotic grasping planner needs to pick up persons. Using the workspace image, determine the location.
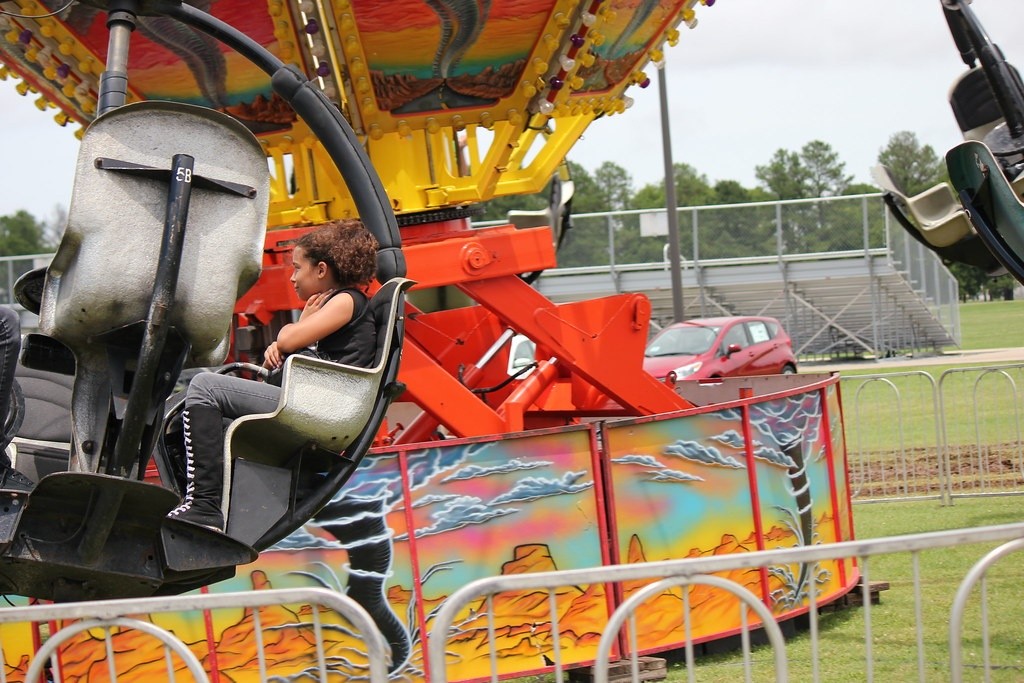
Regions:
[167,219,380,532]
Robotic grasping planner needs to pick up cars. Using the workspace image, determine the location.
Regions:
[508,334,537,380]
[642,314,798,380]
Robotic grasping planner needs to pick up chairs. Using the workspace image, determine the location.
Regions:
[11,101,270,378]
[871,164,975,255]
[948,62,1024,185]
[220,276,419,533]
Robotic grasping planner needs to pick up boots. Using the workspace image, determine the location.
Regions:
[166,405,225,531]
